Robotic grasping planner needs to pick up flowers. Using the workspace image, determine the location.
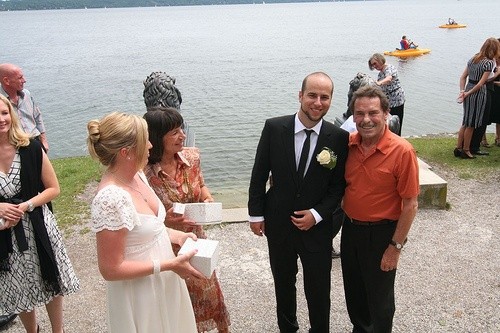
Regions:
[316,146,338,171]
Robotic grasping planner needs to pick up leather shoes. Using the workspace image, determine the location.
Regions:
[471,150,490,155]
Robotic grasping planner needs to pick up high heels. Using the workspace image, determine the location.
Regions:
[453,148,462,157]
[495,138,500,146]
[481,141,490,148]
[459,151,476,159]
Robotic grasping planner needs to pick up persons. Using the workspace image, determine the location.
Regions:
[400,35,417,49]
[0,63,81,333]
[248,72,350,333]
[86,114,207,333]
[341,87,420,333]
[141,107,236,333]
[454,37,500,162]
[448,17,456,24]
[369,53,408,137]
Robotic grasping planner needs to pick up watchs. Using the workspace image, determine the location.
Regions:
[26,200,34,212]
[391,241,403,249]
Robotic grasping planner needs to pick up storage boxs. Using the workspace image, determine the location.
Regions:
[182,203,222,227]
[176,237,220,279]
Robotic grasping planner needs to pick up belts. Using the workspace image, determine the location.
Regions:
[351,218,393,226]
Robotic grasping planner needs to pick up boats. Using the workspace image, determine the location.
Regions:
[384,48,431,60]
[438,24,466,28]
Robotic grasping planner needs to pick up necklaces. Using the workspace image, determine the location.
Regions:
[105,169,147,202]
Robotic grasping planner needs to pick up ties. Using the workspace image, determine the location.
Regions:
[297,129,314,184]
